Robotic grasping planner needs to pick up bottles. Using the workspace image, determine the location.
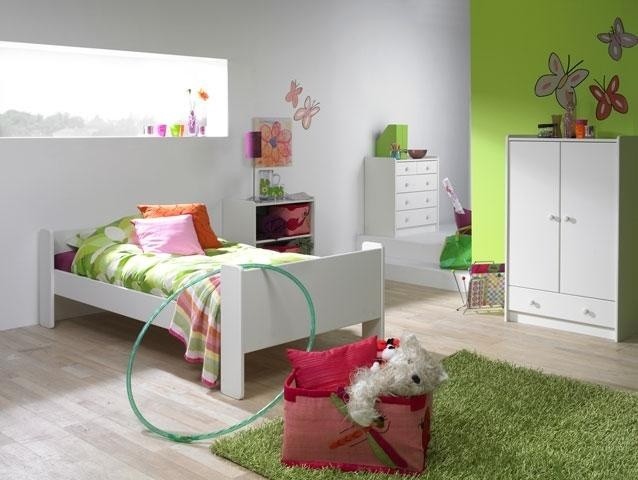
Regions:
[389,143,401,160]
[564,88,575,138]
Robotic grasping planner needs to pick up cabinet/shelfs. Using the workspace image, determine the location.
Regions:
[503,285,638,345]
[506,136,637,301]
[364,154,440,240]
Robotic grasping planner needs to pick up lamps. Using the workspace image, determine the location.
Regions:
[243,130,263,204]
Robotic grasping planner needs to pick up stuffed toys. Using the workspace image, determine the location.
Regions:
[341,335,450,425]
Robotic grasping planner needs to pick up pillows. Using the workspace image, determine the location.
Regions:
[287,336,380,392]
[129,216,206,258]
[136,203,224,250]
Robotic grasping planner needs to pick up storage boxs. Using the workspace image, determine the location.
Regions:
[262,243,301,255]
[281,368,432,473]
[259,202,311,237]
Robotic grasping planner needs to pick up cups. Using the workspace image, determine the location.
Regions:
[573,119,587,139]
[258,169,280,201]
[271,184,288,201]
[142,123,186,138]
[582,125,595,138]
[550,114,563,139]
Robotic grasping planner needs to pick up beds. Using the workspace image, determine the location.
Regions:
[35,215,385,400]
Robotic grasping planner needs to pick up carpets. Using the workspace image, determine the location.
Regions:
[208,349,638,479]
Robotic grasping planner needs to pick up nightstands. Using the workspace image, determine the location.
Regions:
[225,193,315,270]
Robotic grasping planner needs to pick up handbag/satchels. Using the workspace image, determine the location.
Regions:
[440,225,471,270]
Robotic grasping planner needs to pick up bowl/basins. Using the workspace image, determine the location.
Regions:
[408,149,427,160]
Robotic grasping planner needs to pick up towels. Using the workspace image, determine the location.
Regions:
[169,268,223,390]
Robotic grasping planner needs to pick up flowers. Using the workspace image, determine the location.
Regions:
[196,88,208,127]
[258,119,292,163]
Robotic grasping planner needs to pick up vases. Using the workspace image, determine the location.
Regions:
[198,122,207,135]
